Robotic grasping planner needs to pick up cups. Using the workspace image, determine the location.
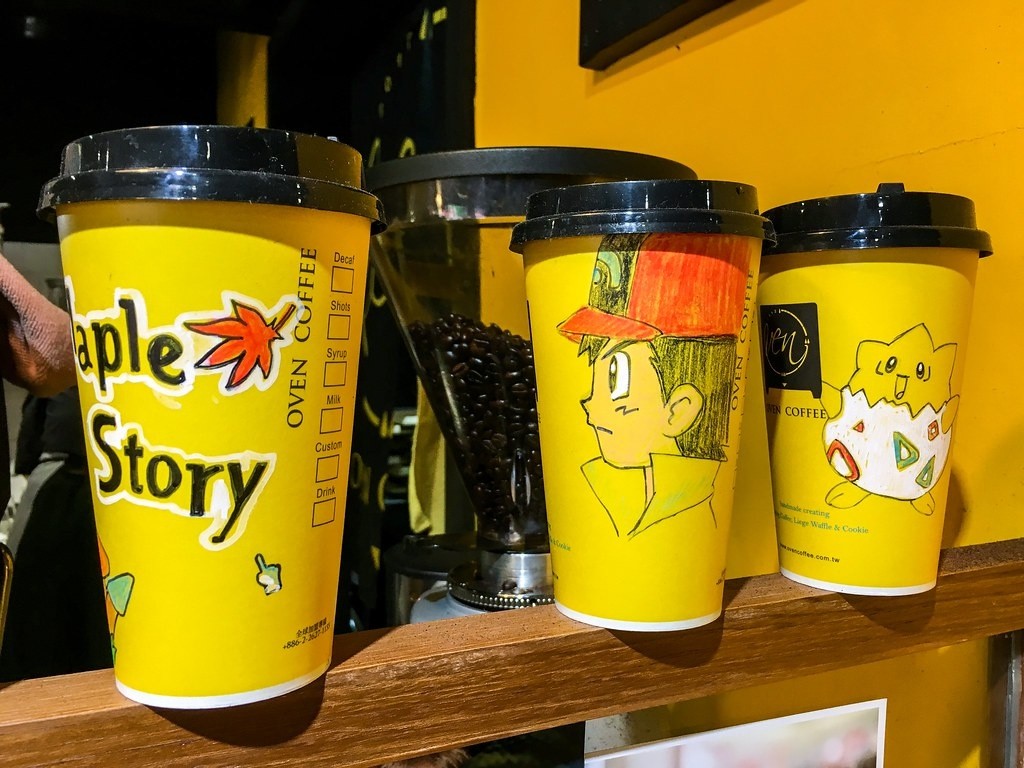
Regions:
[508,181,777,632]
[757,184,994,594]
[34,125,387,708]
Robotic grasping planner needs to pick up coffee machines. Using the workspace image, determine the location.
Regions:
[364,147,700,625]
